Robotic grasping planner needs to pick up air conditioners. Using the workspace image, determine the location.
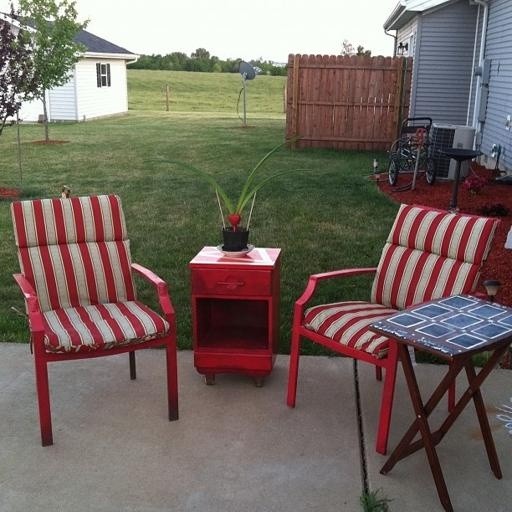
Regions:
[426,123,477,184]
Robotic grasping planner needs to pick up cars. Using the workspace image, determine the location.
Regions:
[252,66,263,76]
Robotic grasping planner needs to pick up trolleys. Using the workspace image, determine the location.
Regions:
[385,114,434,186]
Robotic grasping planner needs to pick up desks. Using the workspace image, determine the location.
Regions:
[366,292,511,510]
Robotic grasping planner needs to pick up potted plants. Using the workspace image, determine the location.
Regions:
[143,135,312,251]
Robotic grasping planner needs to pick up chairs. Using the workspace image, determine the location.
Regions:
[10,195,179,446]
[286,202,503,454]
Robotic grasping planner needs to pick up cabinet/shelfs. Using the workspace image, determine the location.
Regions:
[191,249,283,389]
